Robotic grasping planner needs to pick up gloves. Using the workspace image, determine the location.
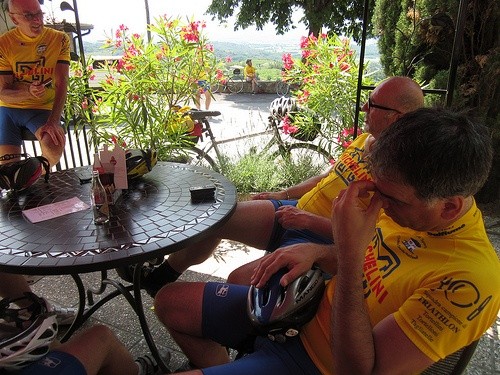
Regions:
[125,149,158,184]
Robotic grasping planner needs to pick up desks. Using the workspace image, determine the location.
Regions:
[0,161,238,375]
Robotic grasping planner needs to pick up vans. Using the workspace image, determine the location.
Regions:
[92,56,123,70]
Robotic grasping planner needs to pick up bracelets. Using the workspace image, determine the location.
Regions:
[283,189,289,200]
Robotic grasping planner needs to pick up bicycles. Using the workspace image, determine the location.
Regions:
[276,71,301,97]
[156,97,336,171]
[205,68,243,94]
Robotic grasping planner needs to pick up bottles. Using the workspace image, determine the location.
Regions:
[90,171,109,225]
[93,154,104,175]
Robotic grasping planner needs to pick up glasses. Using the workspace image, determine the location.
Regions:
[10,11,43,21]
[367,92,401,114]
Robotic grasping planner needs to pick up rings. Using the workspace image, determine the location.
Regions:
[335,196,341,201]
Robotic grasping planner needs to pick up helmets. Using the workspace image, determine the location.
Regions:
[0,292,58,370]
[270,96,296,120]
[247,263,331,344]
[0,154,50,193]
[246,59,251,63]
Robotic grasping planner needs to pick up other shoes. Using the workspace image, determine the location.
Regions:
[251,91,255,95]
[257,84,261,88]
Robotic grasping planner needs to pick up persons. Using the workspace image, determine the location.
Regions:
[115,75,424,299]
[0,0,71,176]
[0,273,172,375]
[153,107,500,375]
[193,48,212,119]
[244,59,262,95]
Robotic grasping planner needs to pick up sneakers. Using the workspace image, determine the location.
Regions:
[134,349,171,375]
[115,263,174,298]
[51,308,76,344]
